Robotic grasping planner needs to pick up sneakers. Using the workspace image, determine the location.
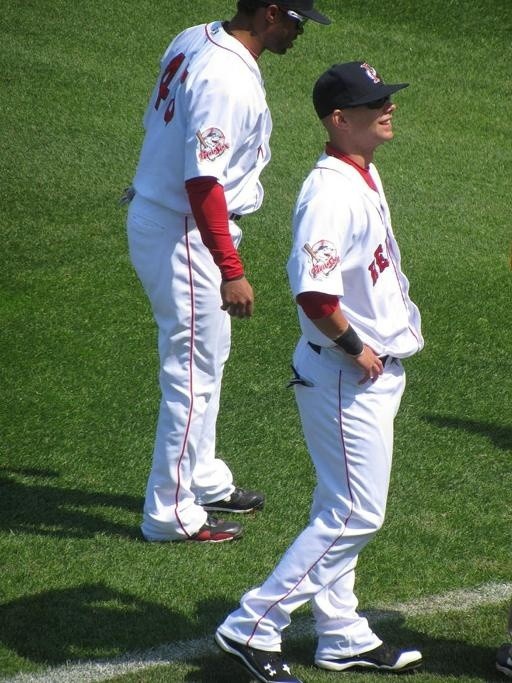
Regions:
[315,644,425,671]
[201,489,266,512]
[189,518,244,542]
[216,632,300,683]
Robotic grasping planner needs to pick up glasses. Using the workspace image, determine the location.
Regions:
[351,99,392,108]
[276,8,308,28]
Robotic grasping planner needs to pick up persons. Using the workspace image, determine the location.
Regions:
[124,0,332,544]
[486,638,511,676]
[215,60,429,681]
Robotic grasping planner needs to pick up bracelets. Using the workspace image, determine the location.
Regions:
[334,326,368,356]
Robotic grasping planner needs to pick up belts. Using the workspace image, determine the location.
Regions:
[231,215,243,223]
[306,341,388,366]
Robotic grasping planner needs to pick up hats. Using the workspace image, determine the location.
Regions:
[290,3,334,27]
[313,62,409,116]
